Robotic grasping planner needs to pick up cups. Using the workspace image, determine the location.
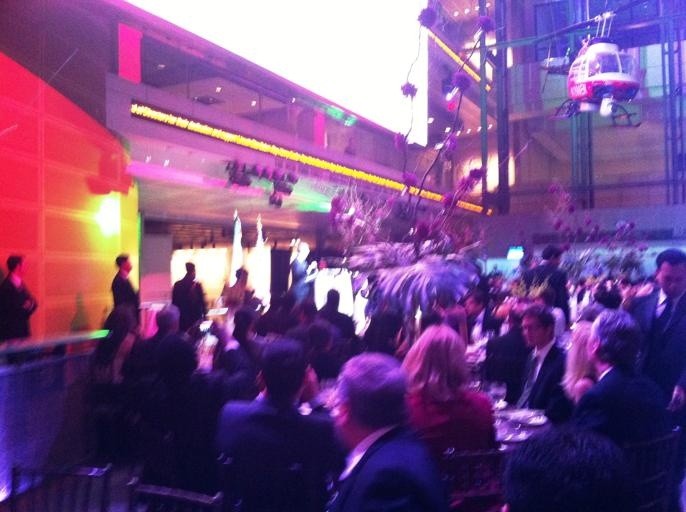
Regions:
[487,330,494,341]
[320,379,336,400]
[491,383,506,417]
[468,377,481,394]
[212,296,225,322]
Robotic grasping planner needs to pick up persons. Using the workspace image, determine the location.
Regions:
[559,321,597,406]
[401,323,506,512]
[92,307,139,417]
[222,269,255,308]
[119,305,198,461]
[142,339,230,495]
[261,290,357,363]
[633,248,686,484]
[502,426,682,512]
[212,307,268,376]
[419,246,656,325]
[505,304,576,428]
[326,352,450,512]
[172,262,209,335]
[288,240,317,304]
[110,255,143,331]
[0,256,39,342]
[218,340,347,512]
[570,309,677,499]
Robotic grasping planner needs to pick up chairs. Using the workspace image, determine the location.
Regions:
[126,476,223,512]
[599,426,680,512]
[442,447,513,512]
[11,462,114,512]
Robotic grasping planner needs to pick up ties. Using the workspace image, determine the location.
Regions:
[527,356,539,384]
[655,299,673,332]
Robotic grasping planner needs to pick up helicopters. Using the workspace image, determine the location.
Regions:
[532,0,644,128]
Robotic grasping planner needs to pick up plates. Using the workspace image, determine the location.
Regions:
[493,398,506,411]
[495,430,529,442]
[508,410,548,426]
